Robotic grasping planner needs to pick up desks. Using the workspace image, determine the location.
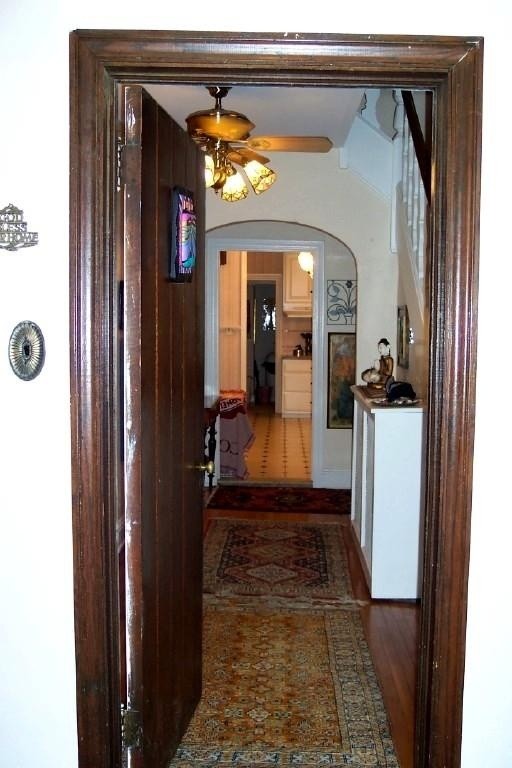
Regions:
[204,392,223,494]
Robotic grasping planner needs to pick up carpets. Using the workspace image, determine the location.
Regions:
[202,482,351,518]
[168,606,399,768]
[200,517,371,608]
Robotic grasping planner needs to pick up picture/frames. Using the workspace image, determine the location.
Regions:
[326,332,357,430]
[169,180,199,284]
[395,305,411,368]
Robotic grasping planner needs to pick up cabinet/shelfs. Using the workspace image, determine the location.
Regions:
[282,251,312,319]
[346,382,428,604]
[280,355,313,417]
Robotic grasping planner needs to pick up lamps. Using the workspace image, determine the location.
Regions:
[202,153,278,203]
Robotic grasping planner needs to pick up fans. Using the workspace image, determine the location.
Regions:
[184,87,334,167]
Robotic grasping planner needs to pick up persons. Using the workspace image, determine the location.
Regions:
[361,337,393,385]
